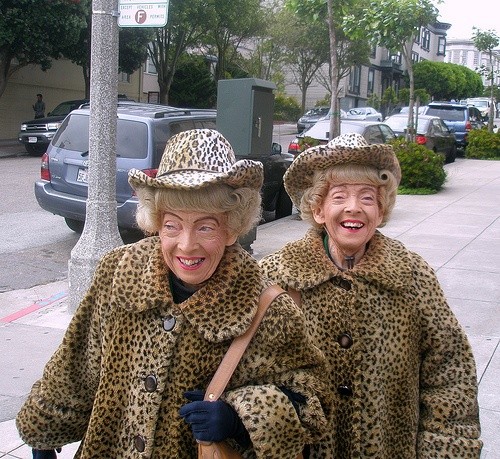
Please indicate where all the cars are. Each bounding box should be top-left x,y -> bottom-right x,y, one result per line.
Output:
297,107 -> 347,134
288,119 -> 398,160
347,107 -> 382,122
382,114 -> 457,164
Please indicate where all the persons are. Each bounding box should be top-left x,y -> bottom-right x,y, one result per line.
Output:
33,93 -> 46,118
15,130 -> 335,459
257,132 -> 483,459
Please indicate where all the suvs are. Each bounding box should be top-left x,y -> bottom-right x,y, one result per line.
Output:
18,94 -> 136,154
34,100 -> 296,236
400,97 -> 500,153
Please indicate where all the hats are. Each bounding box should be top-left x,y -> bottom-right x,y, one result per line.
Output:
127,128 -> 264,191
282,135 -> 401,210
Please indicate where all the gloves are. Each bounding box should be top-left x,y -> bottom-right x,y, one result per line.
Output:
32,443 -> 61,459
179,390 -> 242,443
